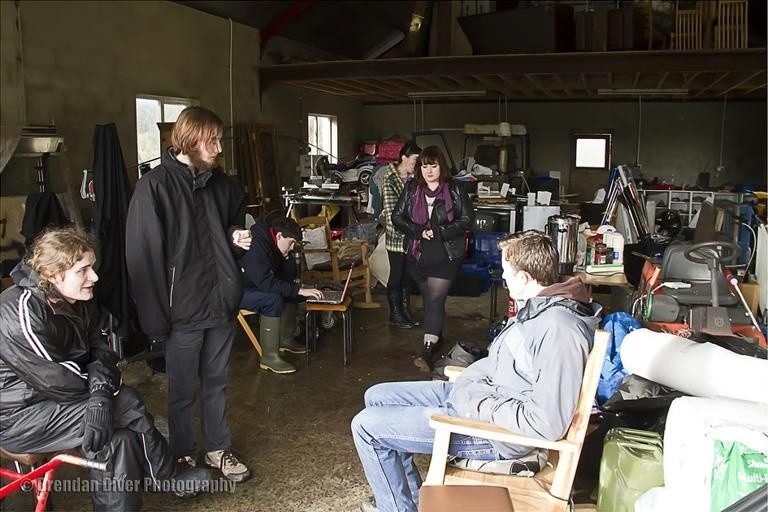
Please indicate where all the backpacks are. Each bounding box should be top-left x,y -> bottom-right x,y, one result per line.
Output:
597,312 -> 642,406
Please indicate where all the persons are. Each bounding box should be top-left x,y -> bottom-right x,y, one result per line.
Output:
0,228 -> 211,512
125,106 -> 252,499
351,229 -> 603,512
382,143 -> 423,329
239,217 -> 325,374
391,146 -> 476,373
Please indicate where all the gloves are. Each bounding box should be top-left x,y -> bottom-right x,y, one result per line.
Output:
144,340 -> 166,373
76,397 -> 115,453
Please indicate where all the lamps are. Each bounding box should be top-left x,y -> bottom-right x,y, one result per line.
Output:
597,87 -> 689,96
407,90 -> 487,98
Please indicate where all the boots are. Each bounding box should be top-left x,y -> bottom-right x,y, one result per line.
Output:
387,287 -> 419,328
260,304 -> 306,373
414,334 -> 439,373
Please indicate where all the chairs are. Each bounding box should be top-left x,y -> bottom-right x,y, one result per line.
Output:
235,309 -> 262,359
421,297 -> 611,512
290,205 -> 382,308
660,244 -> 738,329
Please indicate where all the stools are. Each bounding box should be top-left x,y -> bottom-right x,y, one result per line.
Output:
0,448 -> 64,512
419,484 -> 514,512
301,294 -> 354,367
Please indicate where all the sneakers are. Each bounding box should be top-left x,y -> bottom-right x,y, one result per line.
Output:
148,450 -> 251,497
360,497 -> 376,512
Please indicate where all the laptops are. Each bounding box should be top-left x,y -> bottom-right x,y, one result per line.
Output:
305,261 -> 355,305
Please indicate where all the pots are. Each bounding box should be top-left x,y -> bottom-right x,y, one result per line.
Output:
547,211 -> 579,274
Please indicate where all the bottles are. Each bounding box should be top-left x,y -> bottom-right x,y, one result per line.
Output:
578,221 -> 625,266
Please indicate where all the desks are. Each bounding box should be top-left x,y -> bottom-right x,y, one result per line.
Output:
572,271 -> 628,297
470,201 -> 521,235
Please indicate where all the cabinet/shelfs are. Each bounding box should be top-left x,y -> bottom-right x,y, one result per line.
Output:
636,188 -> 739,230
